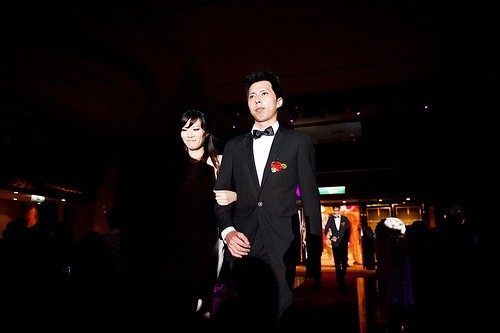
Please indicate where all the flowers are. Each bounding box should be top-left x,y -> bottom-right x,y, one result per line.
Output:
341,219 -> 346,226
269,158 -> 287,173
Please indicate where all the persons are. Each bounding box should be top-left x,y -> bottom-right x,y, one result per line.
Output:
1,180 -> 175,333
210,66 -> 321,333
160,109 -> 239,333
299,199 -> 500,333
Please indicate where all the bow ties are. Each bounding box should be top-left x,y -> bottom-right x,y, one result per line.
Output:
334,216 -> 339,218
253,126 -> 274,139
